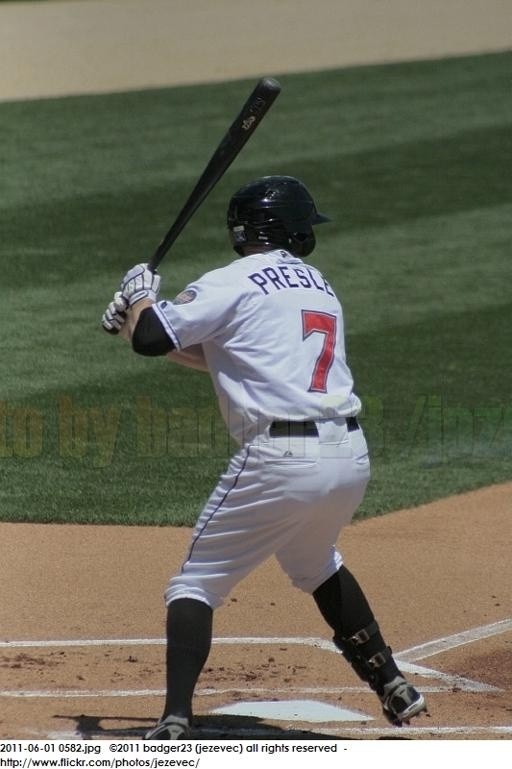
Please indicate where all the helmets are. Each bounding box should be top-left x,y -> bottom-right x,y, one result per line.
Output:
227,175 -> 333,258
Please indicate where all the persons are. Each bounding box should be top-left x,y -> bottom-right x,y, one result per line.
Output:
101,171 -> 426,740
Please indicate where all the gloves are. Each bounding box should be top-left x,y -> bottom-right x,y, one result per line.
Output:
101,262 -> 162,336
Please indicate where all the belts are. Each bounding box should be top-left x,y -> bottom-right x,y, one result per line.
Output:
268,416 -> 359,438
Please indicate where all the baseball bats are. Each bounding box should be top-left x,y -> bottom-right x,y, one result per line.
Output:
102,77 -> 280,335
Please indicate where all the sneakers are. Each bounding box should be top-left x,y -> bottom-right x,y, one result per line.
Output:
379,675 -> 427,726
144,714 -> 192,740
